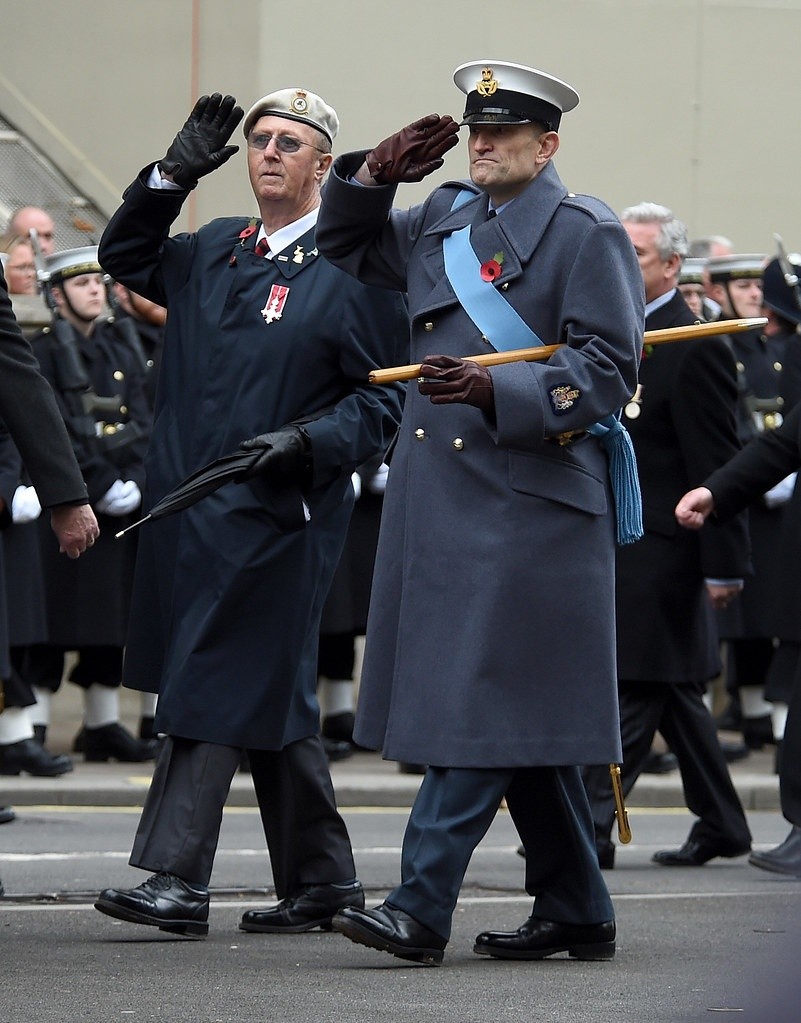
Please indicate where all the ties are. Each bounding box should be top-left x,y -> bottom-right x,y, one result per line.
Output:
485,210 -> 496,221
254,238 -> 271,256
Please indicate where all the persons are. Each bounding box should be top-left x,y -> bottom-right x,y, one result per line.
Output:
97,86 -> 410,938
314,59 -> 650,969
0,197 -> 801,880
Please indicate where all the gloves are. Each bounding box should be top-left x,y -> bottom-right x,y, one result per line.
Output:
233,427 -> 312,487
11,485 -> 41,524
93,478 -> 143,517
417,355 -> 495,413
160,92 -> 245,189
365,113 -> 460,186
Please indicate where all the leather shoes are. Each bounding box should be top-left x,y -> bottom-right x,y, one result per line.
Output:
73,710 -> 380,772
94,872 -> 210,936
517,832 -> 615,868
332,900 -> 447,967
473,914 -> 616,961
0,739 -> 73,776
651,825 -> 751,865
239,883 -> 365,934
713,708 -> 768,761
749,825 -> 801,879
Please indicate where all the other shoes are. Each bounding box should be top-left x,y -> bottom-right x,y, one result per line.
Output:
643,751 -> 676,773
0,808 -> 15,823
398,761 -> 426,775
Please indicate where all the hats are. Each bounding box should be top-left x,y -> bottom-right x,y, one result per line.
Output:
242,86 -> 340,148
677,258 -> 708,284
453,59 -> 580,134
44,245 -> 104,284
708,254 -> 769,281
763,234 -> 801,323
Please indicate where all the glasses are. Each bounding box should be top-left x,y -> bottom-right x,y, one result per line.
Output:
246,132 -> 325,154
